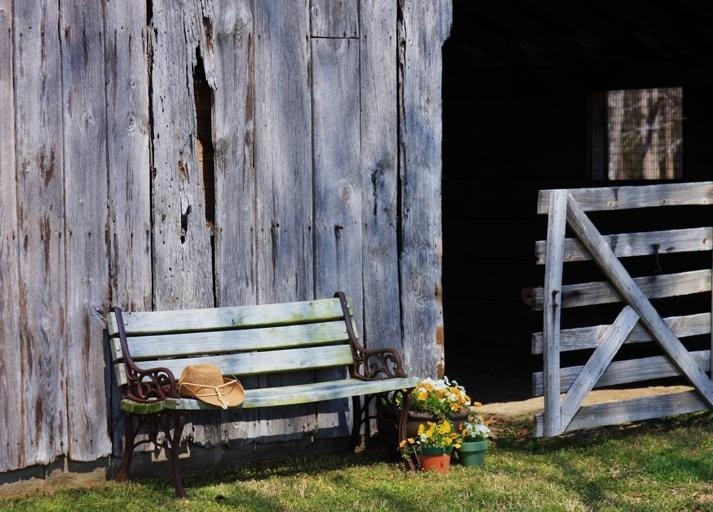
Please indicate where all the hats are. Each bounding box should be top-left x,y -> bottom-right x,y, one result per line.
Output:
162,362 -> 246,410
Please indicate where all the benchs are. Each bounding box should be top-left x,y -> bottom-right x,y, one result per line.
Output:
102,288 -> 421,499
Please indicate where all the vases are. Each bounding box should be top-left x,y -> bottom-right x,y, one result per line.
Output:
415,439 -> 490,475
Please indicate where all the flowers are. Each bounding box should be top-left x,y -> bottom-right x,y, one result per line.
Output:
398,372 -> 496,448
